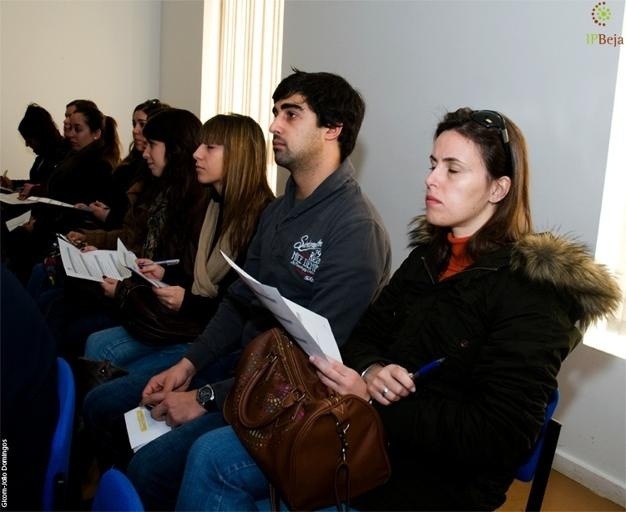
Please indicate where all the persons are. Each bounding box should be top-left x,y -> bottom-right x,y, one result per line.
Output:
1,97 -> 211,378
176,104 -> 622,511
81,70 -> 392,511
44,111 -> 276,486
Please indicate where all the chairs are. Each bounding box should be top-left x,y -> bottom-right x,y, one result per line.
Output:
43,355 -> 78,512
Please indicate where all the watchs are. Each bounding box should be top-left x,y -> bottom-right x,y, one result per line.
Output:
193,381 -> 218,415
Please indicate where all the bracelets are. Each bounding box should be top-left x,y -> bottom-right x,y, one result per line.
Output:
358,361 -> 380,379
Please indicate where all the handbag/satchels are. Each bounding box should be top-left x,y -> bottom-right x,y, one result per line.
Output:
122,293 -> 203,347
225,326 -> 391,511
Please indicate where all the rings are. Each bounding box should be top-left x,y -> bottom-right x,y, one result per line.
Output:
380,386 -> 390,397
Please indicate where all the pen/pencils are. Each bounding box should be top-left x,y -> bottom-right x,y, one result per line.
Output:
139,259 -> 179,266
2,170 -> 7,178
411,358 -> 445,380
104,208 -> 110,209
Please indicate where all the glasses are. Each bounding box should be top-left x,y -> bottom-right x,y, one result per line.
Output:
145,98 -> 161,108
433,110 -> 512,173
